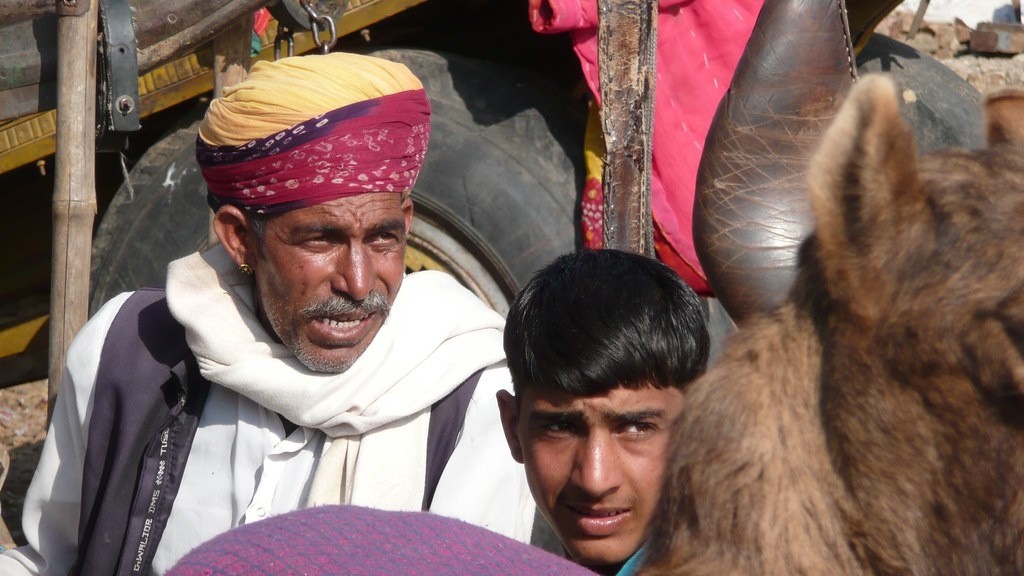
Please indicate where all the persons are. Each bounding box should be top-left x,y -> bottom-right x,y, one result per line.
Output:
494,247 -> 712,575
1,49 -> 573,576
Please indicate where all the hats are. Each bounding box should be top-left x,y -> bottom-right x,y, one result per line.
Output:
196,52 -> 432,219
164,506 -> 601,576
